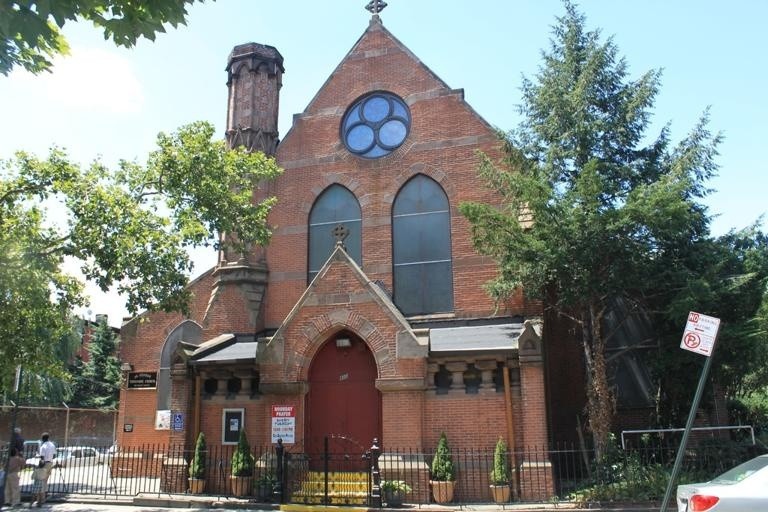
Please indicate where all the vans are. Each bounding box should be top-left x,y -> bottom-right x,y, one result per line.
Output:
2,440 -> 54,467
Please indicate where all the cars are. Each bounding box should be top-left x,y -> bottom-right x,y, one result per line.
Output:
25,447 -> 105,467
95,442 -> 118,454
675,453 -> 767,512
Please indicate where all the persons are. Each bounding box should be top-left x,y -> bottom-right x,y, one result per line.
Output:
28,432 -> 57,509
4,428 -> 26,507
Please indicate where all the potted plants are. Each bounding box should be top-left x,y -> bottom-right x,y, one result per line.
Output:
427,432 -> 456,504
230,426 -> 255,499
255,469 -> 277,499
381,478 -> 411,506
187,432 -> 207,494
489,437 -> 511,504
